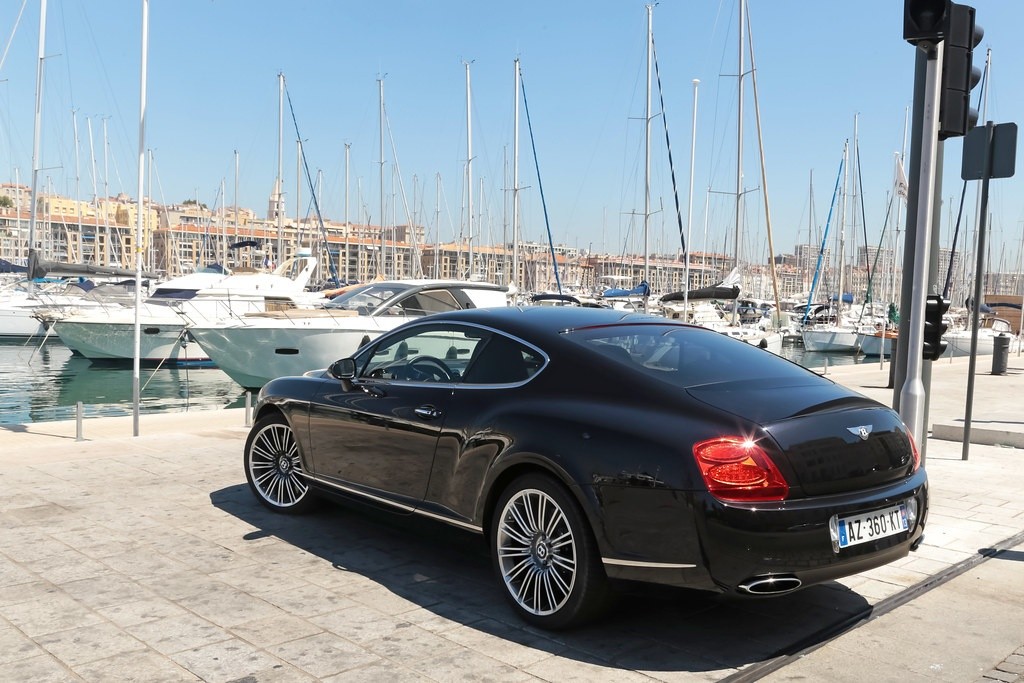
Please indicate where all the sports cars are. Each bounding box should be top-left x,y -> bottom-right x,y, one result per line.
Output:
240,306 -> 935,631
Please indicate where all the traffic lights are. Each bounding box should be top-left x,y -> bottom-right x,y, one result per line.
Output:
902,0 -> 949,41
940,4 -> 985,142
924,295 -> 951,361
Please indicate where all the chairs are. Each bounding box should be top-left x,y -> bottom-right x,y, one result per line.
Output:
463,340 -> 529,386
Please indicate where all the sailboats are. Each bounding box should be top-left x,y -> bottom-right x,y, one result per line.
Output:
0,0 -> 1024,391
